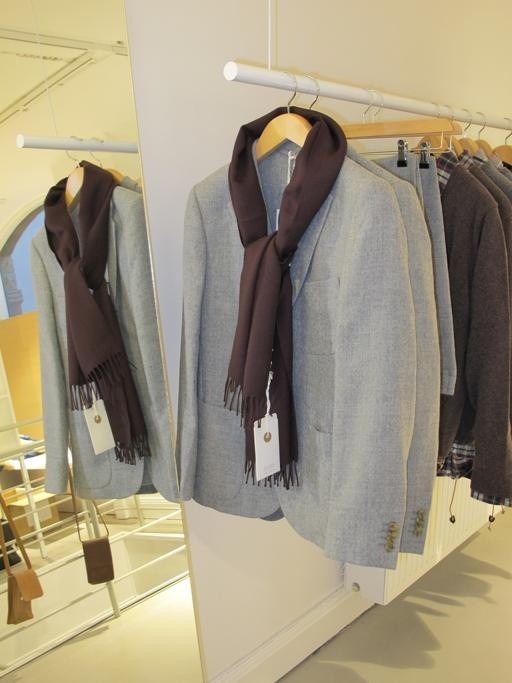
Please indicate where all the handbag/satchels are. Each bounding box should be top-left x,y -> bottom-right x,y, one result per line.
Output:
7,569 -> 43,625
83,537 -> 114,585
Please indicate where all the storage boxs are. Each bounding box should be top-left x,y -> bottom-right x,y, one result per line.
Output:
3,489 -> 60,537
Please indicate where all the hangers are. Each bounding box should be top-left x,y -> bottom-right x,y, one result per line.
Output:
64,135 -> 143,208
250,68 -> 511,169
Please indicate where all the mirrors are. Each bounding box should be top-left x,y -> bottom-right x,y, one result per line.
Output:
0,0 -> 204,683
